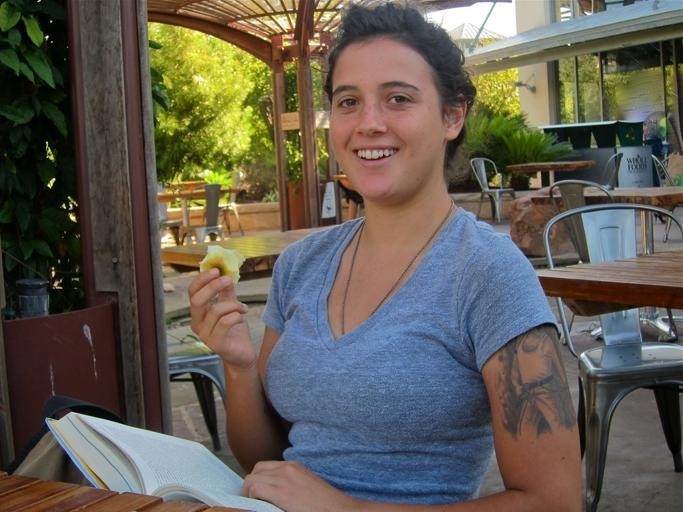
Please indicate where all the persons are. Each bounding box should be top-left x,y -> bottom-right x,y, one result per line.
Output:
185,5 -> 584,511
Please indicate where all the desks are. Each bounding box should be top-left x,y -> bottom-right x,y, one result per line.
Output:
527,182 -> 682,254
535,248 -> 683,319
506,160 -> 682,343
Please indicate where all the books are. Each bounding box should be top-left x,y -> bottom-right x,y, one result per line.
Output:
40,411 -> 282,511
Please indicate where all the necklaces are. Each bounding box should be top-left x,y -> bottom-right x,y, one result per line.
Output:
338,195 -> 455,341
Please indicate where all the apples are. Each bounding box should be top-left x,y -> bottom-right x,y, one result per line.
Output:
199,244 -> 244,285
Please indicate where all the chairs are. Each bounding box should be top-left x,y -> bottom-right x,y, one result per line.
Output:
602,153 -> 675,244
157,172 -> 245,246
470,157 -> 517,224
546,177 -> 618,260
544,202 -> 680,509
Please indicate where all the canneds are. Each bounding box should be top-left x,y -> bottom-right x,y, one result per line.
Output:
16,278 -> 51,317
